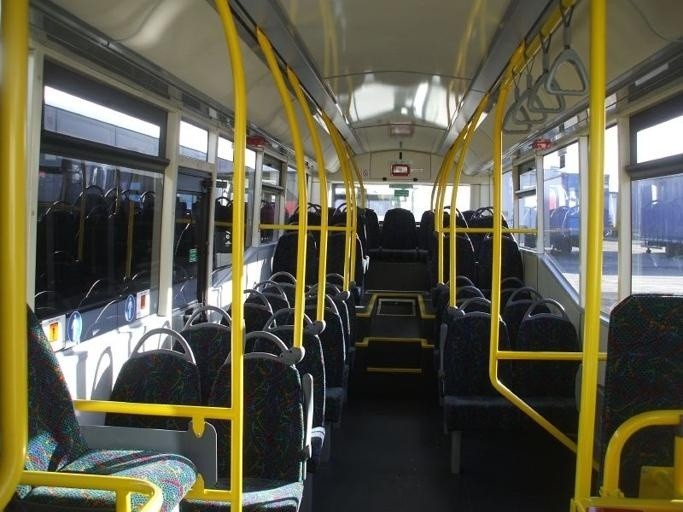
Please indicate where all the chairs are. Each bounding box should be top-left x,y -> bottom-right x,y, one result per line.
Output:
420,206 -> 524,315
380,207 -> 417,263
433,276 -> 582,498
600,292 -> 683,497
9,304 -> 197,510
106,271 -> 373,512
273,203 -> 379,314
